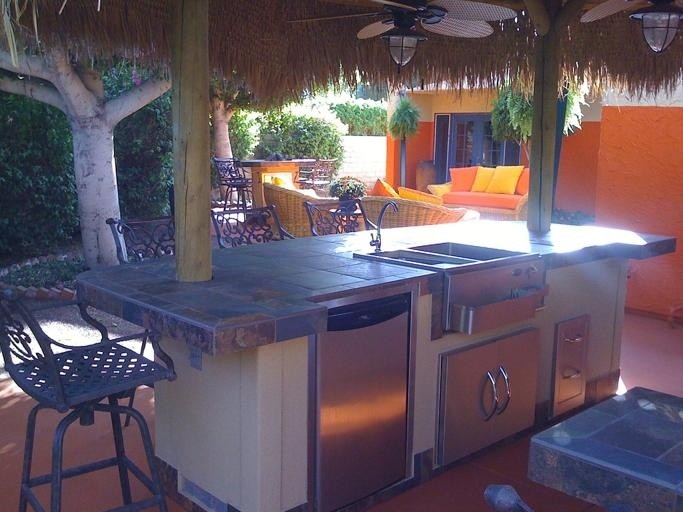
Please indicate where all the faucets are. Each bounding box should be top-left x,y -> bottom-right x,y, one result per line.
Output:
370,202 -> 399,250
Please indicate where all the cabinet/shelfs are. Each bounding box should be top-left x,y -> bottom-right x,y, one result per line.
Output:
436,327 -> 537,471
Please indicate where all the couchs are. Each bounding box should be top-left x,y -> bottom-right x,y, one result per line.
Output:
429,164 -> 531,224
362,190 -> 468,228
266,176 -> 339,237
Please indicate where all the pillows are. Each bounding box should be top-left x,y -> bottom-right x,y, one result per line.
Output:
368,178 -> 400,200
447,166 -> 480,194
398,186 -> 445,204
472,167 -> 495,194
489,167 -> 524,195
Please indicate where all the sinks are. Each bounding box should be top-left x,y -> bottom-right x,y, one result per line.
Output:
409,241 -> 539,263
352,249 -> 481,271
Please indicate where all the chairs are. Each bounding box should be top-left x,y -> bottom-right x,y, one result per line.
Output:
211,203 -> 297,250
216,157 -> 255,225
2,280 -> 177,507
105,215 -> 176,265
299,159 -> 337,195
302,198 -> 380,235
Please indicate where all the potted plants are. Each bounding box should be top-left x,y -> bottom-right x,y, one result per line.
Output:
332,175 -> 367,211
